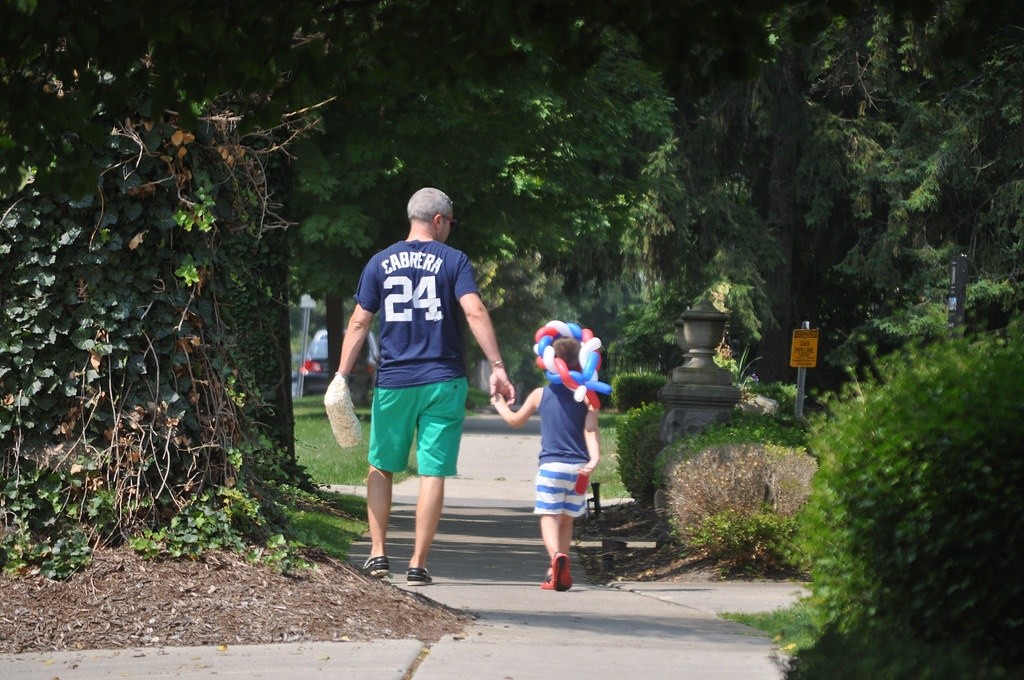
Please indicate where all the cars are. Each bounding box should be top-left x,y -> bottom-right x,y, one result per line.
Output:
294,326 -> 378,393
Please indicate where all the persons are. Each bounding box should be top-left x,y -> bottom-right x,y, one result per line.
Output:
490,337 -> 600,593
324,188 -> 516,586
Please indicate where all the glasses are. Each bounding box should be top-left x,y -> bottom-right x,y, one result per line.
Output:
432,214 -> 458,228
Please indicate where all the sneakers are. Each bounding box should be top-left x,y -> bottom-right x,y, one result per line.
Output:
541,569 -> 554,590
552,552 -> 572,591
363,555 -> 390,576
406,567 -> 431,586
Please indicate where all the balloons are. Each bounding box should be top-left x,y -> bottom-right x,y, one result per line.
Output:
534,321 -> 612,408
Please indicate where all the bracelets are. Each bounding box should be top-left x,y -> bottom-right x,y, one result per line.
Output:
334,371 -> 350,382
490,360 -> 504,368
